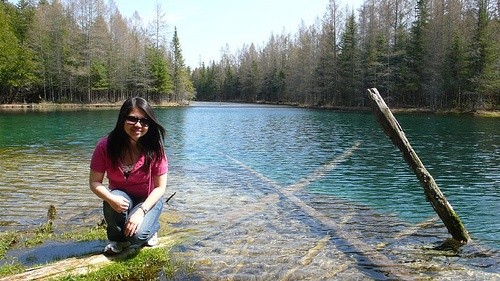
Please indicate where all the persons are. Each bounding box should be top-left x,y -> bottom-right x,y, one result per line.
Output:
89,97 -> 168,255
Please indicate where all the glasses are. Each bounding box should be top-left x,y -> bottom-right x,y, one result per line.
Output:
125,116 -> 150,127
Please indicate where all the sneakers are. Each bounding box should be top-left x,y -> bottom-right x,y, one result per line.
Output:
104,241 -> 131,253
145,232 -> 158,246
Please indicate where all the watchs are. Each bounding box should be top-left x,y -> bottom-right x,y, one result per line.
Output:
139,204 -> 148,214
